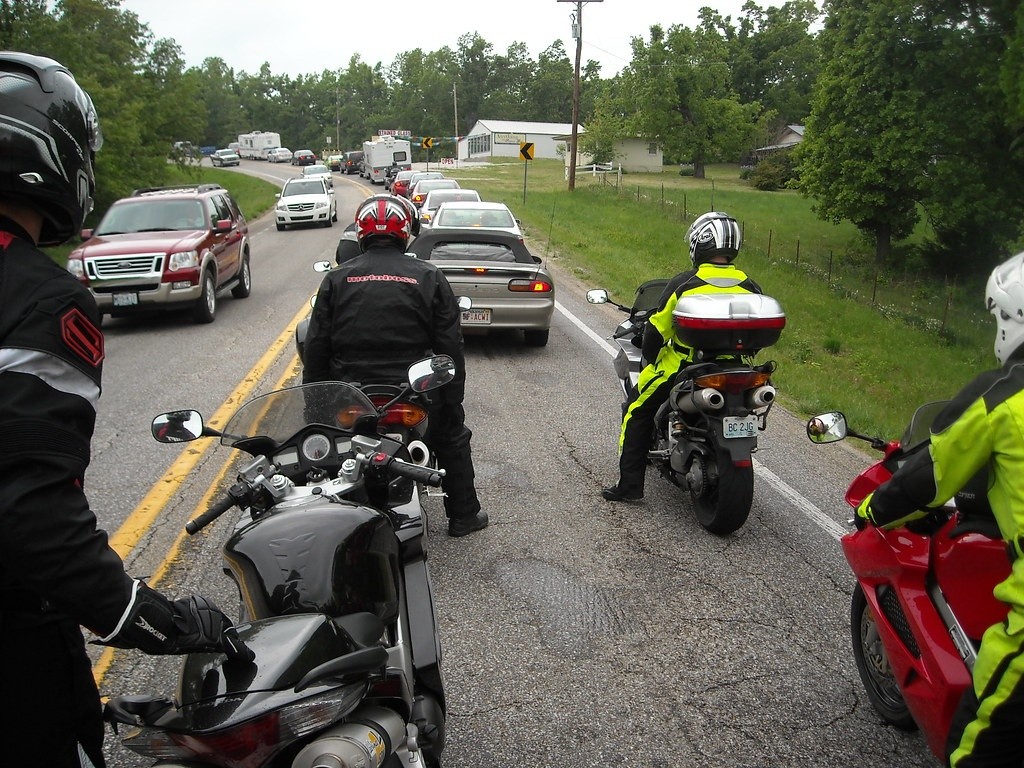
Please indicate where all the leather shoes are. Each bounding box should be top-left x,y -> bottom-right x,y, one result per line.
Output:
448,510 -> 487,537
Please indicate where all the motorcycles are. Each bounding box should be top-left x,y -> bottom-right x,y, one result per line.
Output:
308,253 -> 471,466
96,349 -> 465,767
807,397 -> 1016,768
584,282 -> 781,536
383,167 -> 403,190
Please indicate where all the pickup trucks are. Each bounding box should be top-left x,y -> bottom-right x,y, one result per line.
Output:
209,149 -> 240,166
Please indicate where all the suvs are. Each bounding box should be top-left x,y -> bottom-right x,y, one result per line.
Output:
68,183 -> 251,324
274,176 -> 338,230
339,151 -> 365,175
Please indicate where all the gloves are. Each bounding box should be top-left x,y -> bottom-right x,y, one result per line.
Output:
87,577 -> 240,656
854,489 -> 875,532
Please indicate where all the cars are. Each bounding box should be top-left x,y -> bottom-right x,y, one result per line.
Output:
422,201 -> 525,245
405,229 -> 555,347
291,150 -> 316,166
389,170 -> 444,195
409,179 -> 462,208
267,147 -> 293,163
227,142 -> 239,156
418,190 -> 482,225
323,155 -> 343,171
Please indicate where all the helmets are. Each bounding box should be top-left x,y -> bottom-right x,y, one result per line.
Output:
683,212 -> 741,269
984,252 -> 1024,366
0,50 -> 104,248
354,194 -> 413,255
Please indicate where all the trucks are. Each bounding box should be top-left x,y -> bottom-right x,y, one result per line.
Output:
357,135 -> 412,183
174,141 -> 192,156
238,131 -> 281,160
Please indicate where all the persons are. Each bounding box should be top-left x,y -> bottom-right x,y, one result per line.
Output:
602,210 -> 763,503
152,411 -> 196,441
410,357 -> 455,390
0,50 -> 286,768
301,194 -> 489,537
809,418 -> 839,443
852,251 -> 1024,767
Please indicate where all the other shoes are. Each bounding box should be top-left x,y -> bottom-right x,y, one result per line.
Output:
602,484 -> 644,502
